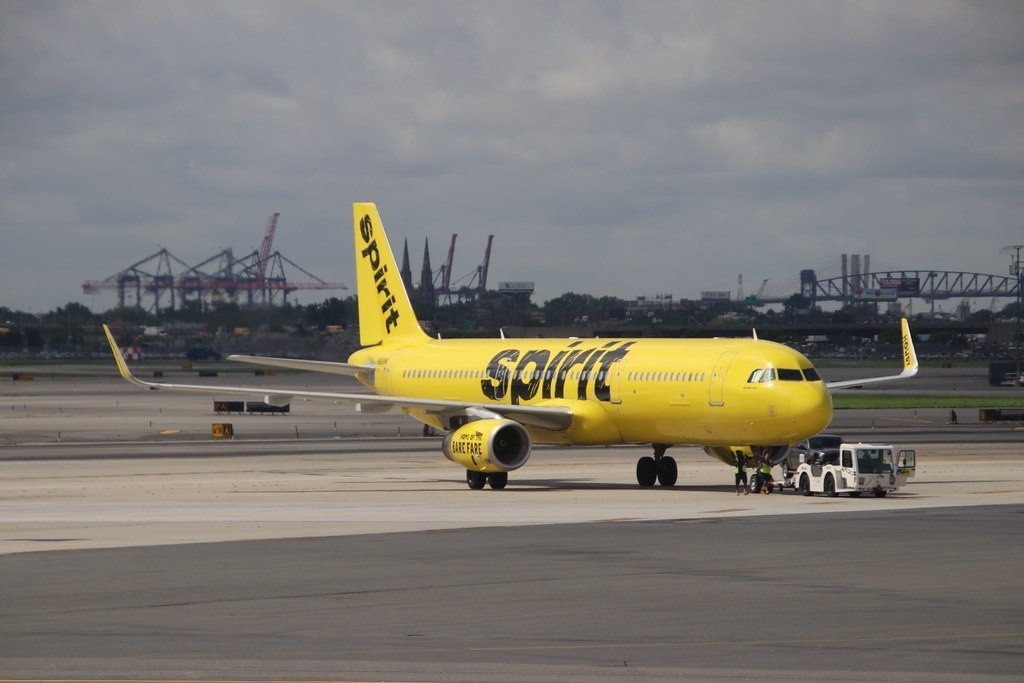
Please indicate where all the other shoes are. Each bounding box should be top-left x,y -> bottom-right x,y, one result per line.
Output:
743,489 -> 748,495
736,490 -> 739,495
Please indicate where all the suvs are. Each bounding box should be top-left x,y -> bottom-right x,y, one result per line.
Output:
779,436 -> 844,480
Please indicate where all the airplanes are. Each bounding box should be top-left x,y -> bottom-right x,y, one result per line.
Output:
103,202 -> 918,490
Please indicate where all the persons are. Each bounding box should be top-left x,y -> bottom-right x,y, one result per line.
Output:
734,451 -> 749,495
755,454 -> 773,495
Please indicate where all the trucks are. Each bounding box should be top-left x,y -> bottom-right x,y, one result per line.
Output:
796,444 -> 916,497
